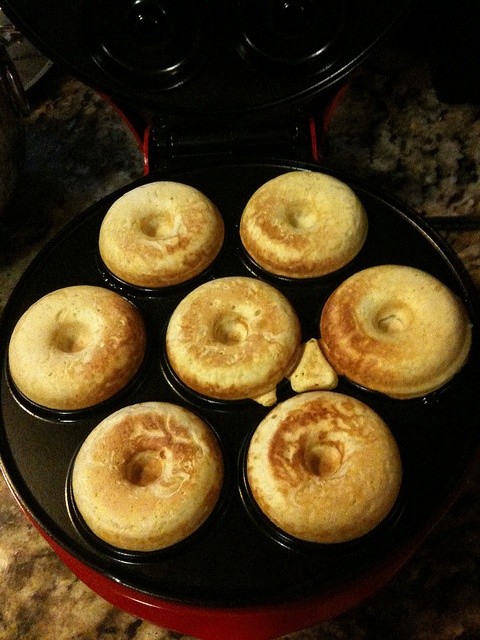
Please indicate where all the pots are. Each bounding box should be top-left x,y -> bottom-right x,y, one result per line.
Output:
3,2 -> 477,632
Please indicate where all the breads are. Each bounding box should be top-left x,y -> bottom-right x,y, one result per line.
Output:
71,400 -> 221,552
320,264 -> 470,398
8,285 -> 144,409
99,180 -> 224,289
246,389 -> 403,545
239,169 -> 368,277
166,275 -> 301,400
